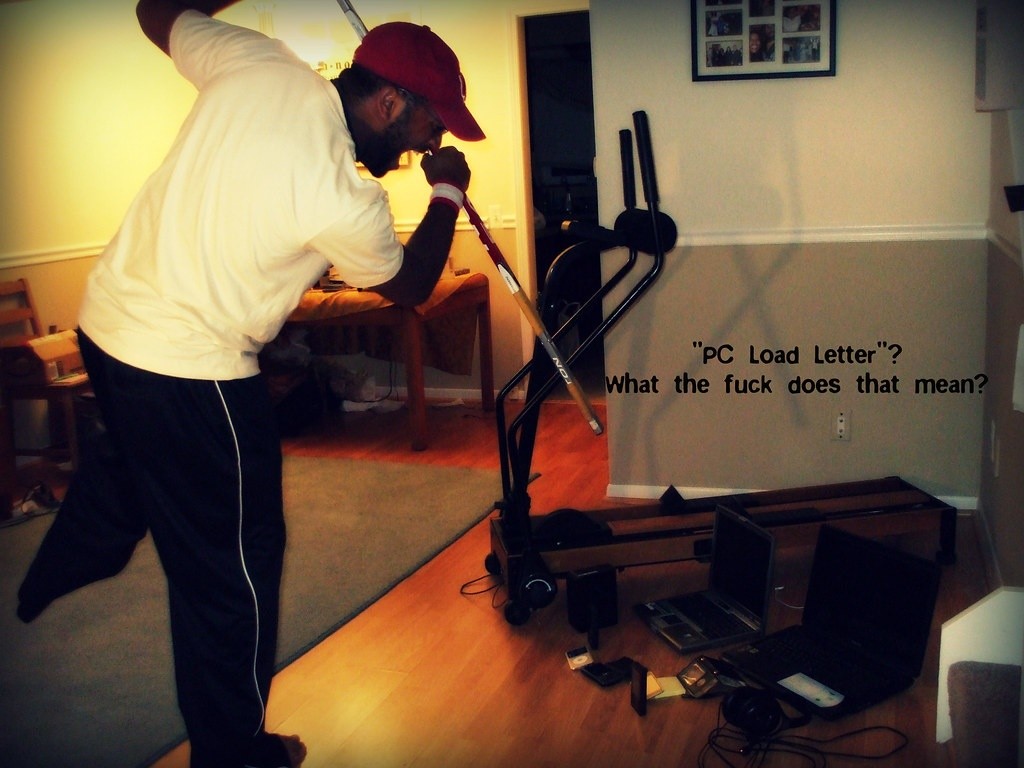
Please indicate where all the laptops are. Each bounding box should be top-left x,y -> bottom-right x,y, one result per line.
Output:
631,503 -> 776,654
720,522 -> 942,721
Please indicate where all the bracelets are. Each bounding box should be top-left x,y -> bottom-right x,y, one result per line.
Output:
430,182 -> 464,209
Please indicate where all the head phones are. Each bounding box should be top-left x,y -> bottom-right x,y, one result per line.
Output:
721,685 -> 813,738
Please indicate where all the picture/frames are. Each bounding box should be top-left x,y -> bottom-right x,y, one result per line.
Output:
690,0 -> 837,82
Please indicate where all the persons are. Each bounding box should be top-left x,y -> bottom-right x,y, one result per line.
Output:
19,1 -> 486,767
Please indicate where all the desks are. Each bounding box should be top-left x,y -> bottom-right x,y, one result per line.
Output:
277,271 -> 495,451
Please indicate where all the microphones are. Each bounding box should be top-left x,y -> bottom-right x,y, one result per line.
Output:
740,739 -> 762,756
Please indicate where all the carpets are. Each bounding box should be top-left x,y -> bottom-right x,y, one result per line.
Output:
0,453 -> 543,768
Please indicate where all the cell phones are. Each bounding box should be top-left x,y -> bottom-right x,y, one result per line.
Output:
582,662 -> 624,687
631,662 -> 647,715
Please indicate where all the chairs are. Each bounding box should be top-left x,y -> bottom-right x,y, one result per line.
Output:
0,276 -> 91,507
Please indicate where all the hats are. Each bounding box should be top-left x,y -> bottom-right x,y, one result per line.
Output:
351,22 -> 486,144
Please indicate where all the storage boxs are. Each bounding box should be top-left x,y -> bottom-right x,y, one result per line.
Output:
0,330 -> 84,391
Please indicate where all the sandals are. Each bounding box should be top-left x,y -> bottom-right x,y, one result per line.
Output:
187,729 -> 310,768
